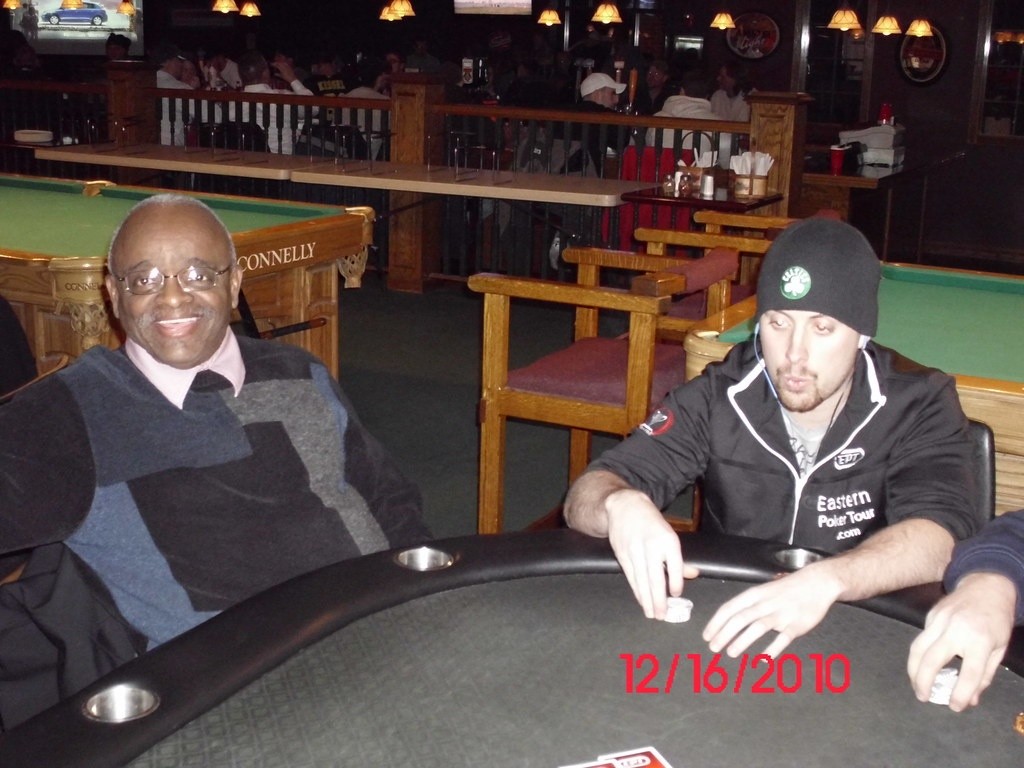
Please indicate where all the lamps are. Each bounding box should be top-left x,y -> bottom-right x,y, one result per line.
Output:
205,0 -> 933,42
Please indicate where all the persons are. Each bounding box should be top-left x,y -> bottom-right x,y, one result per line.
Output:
711,60 -> 754,156
103,26 -> 458,163
0,194 -> 434,734
0,3 -> 102,179
562,218 -> 980,663
440,37 -> 712,183
907,509 -> 1024,714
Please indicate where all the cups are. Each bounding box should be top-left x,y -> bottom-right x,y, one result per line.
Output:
829,147 -> 845,175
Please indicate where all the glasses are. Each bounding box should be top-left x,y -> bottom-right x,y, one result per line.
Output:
645,71 -> 665,80
106,262 -> 232,296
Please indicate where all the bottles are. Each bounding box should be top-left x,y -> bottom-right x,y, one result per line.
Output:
879,102 -> 893,121
679,175 -> 692,199
235,80 -> 240,91
223,81 -> 228,90
662,175 -> 675,196
699,174 -> 714,201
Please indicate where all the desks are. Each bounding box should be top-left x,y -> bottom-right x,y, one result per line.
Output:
2,167 -> 375,394
0,527 -> 997,768
802,147 -> 906,264
36,142 -> 660,210
680,253 -> 1024,513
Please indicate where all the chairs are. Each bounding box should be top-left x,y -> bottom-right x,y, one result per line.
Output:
631,205 -> 842,345
466,247 -> 739,539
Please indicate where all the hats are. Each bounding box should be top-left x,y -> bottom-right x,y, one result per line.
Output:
580,73 -> 626,98
753,217 -> 882,338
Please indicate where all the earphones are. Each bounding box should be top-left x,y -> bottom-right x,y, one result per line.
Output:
754,321 -> 760,333
861,340 -> 867,352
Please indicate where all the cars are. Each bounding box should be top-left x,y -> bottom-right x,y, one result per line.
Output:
40,1 -> 108,27
